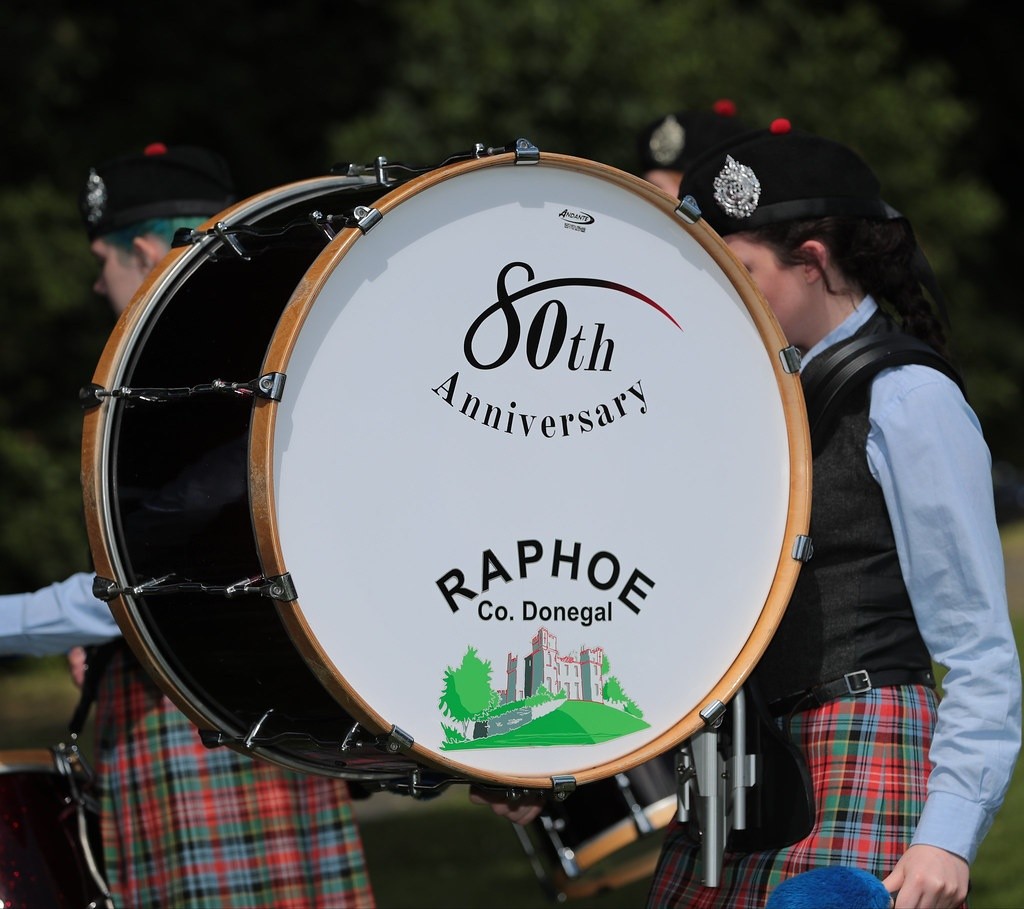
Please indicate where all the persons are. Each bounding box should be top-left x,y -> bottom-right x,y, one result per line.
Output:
638,98 -> 749,202
467,128 -> 1023,908
0,138 -> 374,909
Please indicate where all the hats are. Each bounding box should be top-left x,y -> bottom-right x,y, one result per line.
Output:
630,99 -> 741,171
78,141 -> 227,241
678,121 -> 881,236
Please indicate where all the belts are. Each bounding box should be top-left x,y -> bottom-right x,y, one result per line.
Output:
769,669 -> 936,719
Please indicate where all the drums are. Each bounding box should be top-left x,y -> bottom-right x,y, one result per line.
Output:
509,750 -> 684,898
1,744 -> 128,909
69,138 -> 823,799
